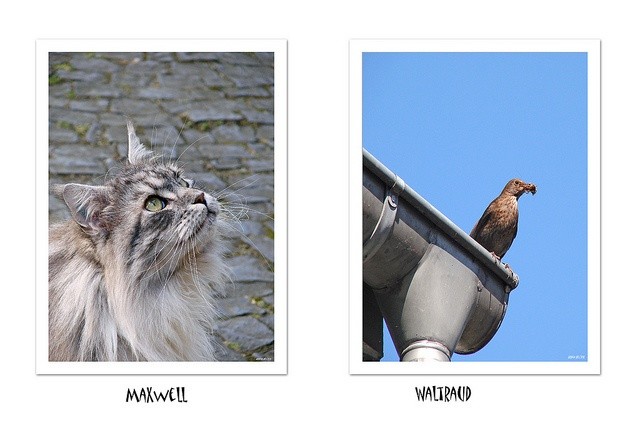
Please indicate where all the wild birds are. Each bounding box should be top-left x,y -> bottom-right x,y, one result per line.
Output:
469,179 -> 538,277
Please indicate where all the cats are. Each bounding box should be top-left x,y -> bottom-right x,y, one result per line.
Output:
49,118 -> 228,361
